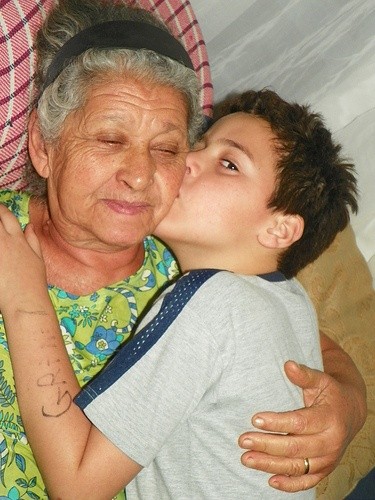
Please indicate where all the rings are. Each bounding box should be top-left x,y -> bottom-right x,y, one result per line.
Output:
304,457 -> 310,474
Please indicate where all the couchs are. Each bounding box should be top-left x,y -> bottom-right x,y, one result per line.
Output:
1,0 -> 374,497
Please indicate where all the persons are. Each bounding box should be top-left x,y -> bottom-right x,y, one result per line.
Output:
1,85 -> 357,499
0,1 -> 366,498
